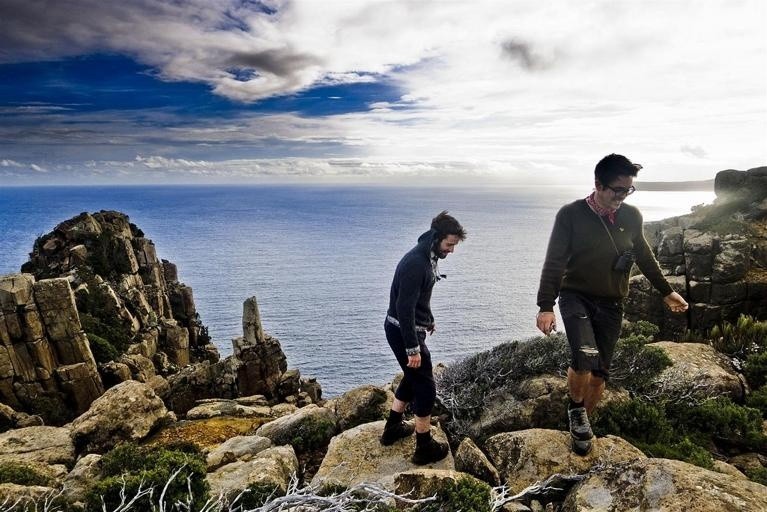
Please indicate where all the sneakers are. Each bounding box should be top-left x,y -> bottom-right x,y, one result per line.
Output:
567,403 -> 596,456
381,420 -> 416,446
411,436 -> 449,465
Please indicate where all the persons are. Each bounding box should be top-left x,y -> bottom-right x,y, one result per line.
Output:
537,153 -> 690,455
380,209 -> 466,464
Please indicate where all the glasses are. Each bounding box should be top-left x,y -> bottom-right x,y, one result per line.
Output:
605,183 -> 635,198
